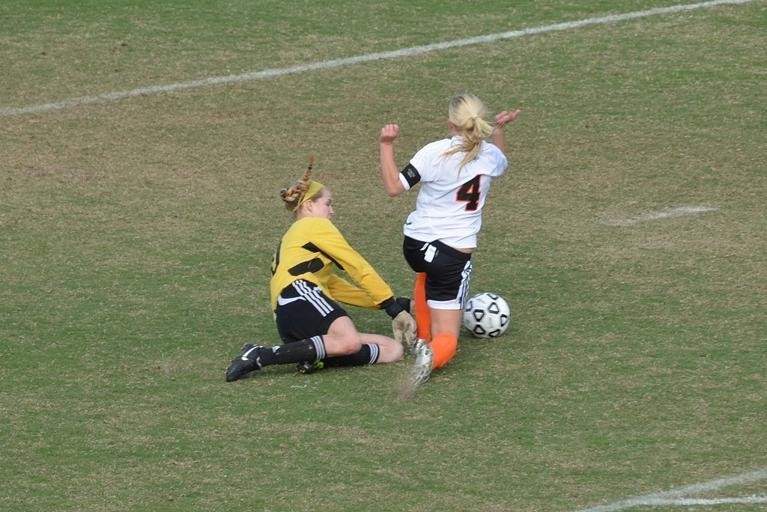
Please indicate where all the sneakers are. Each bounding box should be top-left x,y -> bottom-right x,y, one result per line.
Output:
226,343 -> 265,382
295,359 -> 327,374
413,339 -> 433,386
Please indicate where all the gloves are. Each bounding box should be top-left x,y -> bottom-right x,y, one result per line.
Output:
392,296 -> 418,352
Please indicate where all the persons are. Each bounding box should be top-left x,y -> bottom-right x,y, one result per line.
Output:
379,93 -> 521,385
226,154 -> 418,382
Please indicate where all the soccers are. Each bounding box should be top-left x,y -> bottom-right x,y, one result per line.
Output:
464,291 -> 511,337
